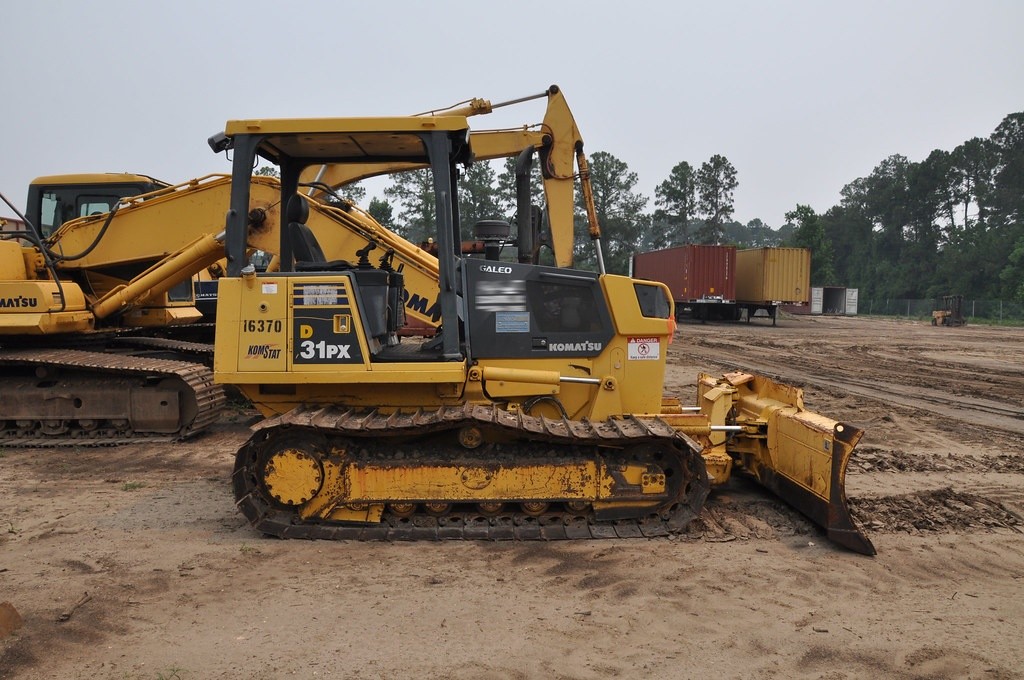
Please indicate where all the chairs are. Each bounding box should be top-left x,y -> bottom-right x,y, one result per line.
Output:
285,192 -> 357,271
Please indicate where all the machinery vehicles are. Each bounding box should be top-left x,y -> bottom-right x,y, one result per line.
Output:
931,295 -> 967,327
207,85 -> 880,557
0,174 -> 439,446
